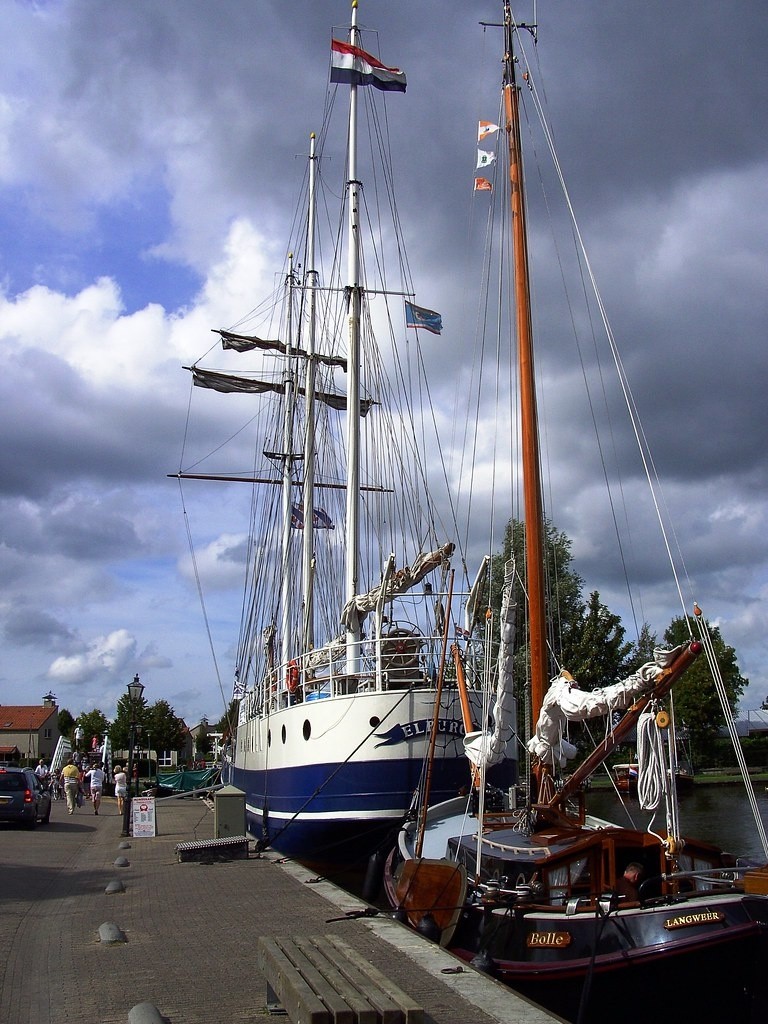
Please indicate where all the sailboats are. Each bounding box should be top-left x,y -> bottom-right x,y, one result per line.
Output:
166,0 -> 522,887
381,0 -> 768,1010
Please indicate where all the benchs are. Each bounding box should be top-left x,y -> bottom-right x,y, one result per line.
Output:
177,836 -> 250,863
257,933 -> 423,1024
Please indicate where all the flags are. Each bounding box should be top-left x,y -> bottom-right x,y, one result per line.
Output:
474,178 -> 492,191
291,501 -> 335,530
457,627 -> 470,641
479,121 -> 499,141
629,766 -> 639,777
330,39 -> 407,94
476,148 -> 495,169
405,299 -> 443,336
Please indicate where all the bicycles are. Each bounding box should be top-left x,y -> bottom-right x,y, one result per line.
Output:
50,774 -> 59,801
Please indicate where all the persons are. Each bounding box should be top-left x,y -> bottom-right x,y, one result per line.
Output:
82,750 -> 90,764
614,862 -> 643,903
72,748 -> 82,764
133,764 -> 137,782
60,759 -> 79,815
51,764 -> 91,800
85,764 -> 104,815
113,765 -> 126,815
74,724 -> 84,747
34,759 -> 48,776
91,734 -> 98,748
186,758 -> 206,770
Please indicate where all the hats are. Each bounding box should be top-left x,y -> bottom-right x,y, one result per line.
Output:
113,765 -> 121,772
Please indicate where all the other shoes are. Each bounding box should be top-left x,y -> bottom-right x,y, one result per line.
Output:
68,810 -> 73,815
54,797 -> 57,800
120,812 -> 123,815
94,811 -> 98,815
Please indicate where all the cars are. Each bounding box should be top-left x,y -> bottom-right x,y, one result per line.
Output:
0,766 -> 52,829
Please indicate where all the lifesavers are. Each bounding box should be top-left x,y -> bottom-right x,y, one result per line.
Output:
285,660 -> 300,692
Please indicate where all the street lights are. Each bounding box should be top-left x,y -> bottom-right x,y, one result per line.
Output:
145,729 -> 155,780
135,722 -> 142,797
118,672 -> 145,839
